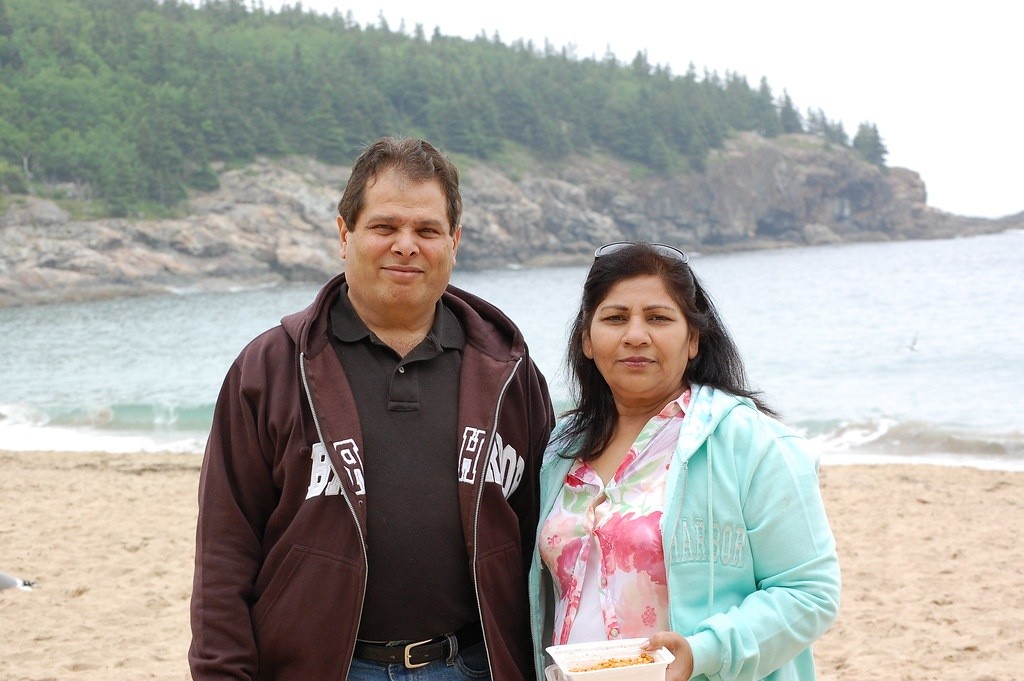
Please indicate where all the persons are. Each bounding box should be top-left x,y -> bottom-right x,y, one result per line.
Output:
188,137 -> 557,681
523,240 -> 843,681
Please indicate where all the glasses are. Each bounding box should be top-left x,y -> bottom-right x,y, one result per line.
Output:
594,239 -> 690,268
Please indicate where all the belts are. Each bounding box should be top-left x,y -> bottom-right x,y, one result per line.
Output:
353,621 -> 484,669
